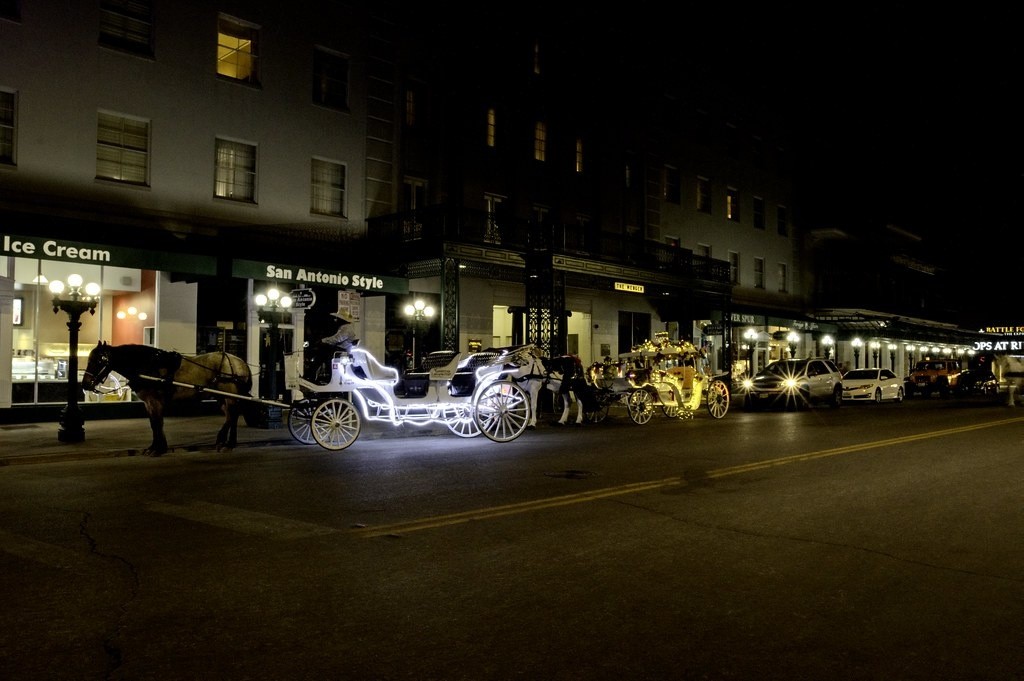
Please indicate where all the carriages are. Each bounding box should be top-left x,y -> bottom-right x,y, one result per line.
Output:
81,335 -> 536,457
509,332 -> 731,431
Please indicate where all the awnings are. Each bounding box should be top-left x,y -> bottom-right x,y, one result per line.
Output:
723,301 -> 838,333
227,253 -> 409,294
0,223 -> 216,276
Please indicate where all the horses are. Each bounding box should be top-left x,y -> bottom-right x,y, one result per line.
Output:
81,340 -> 253,457
508,352 -> 589,430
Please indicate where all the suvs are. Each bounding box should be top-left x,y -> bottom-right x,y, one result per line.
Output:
907,359 -> 963,400
744,357 -> 844,413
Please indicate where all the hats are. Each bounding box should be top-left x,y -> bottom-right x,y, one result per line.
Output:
330,307 -> 353,323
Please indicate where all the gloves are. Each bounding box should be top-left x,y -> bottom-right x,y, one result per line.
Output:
314,339 -> 322,345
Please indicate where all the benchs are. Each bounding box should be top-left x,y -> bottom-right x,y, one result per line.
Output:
350,350 -> 399,385
449,351 -> 506,397
401,351 -> 460,397
665,366 -> 695,400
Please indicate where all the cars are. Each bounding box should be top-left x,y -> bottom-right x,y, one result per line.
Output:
840,367 -> 906,406
961,367 -> 1000,397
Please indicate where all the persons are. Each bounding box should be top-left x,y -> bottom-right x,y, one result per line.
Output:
322,308 -> 359,348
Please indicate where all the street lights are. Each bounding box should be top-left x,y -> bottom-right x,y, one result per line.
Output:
400,298 -> 436,373
919,346 -> 929,361
871,342 -> 881,369
744,327 -> 758,379
820,335 -> 835,360
254,287 -> 293,429
49,265 -> 101,446
787,331 -> 799,358
888,343 -> 898,373
906,344 -> 915,373
851,338 -> 862,369
